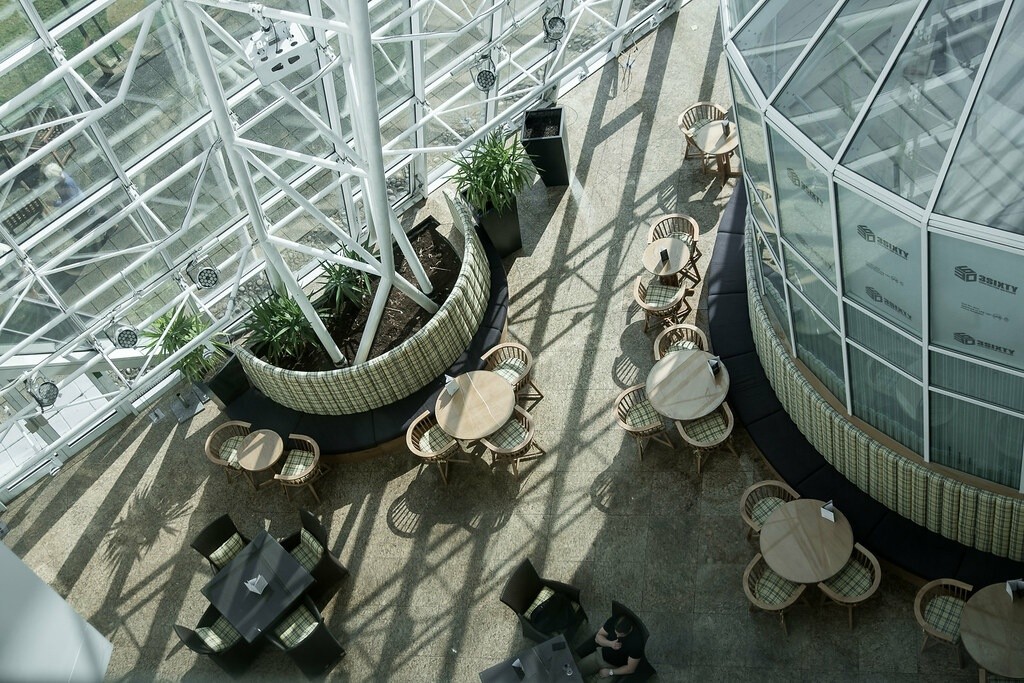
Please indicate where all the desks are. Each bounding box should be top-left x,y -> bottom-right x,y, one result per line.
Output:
760,500 -> 853,584
960,582 -> 1024,680
697,119 -> 743,190
645,350 -> 729,421
479,634 -> 584,683
434,370 -> 514,440
641,238 -> 694,313
238,428 -> 284,490
200,529 -> 314,644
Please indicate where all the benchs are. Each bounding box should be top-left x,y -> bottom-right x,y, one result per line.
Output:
703,172 -> 1023,591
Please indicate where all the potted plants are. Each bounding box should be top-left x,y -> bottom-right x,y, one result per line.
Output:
137,305 -> 252,420
443,121 -> 546,259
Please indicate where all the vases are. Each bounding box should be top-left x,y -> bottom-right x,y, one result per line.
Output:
520,108 -> 571,187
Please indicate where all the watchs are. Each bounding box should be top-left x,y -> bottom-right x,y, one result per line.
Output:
609,669 -> 613,675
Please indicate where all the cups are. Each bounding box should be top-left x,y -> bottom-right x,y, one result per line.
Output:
563,663 -> 573,676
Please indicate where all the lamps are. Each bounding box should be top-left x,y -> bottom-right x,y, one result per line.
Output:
185,253 -> 222,291
102,315 -> 139,349
27,369 -> 60,408
469,52 -> 498,91
543,2 -> 566,45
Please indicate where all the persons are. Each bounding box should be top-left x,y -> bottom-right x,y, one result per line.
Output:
44,163 -> 118,252
579,616 -> 644,683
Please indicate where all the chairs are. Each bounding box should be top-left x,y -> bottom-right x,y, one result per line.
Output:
614,212 -> 887,640
406,344 -> 546,487
205,420 -> 337,506
579,600 -> 657,683
677,101 -> 735,172
914,578 -> 1024,683
173,514 -> 350,681
0,106 -> 76,235
499,559 -> 588,648
